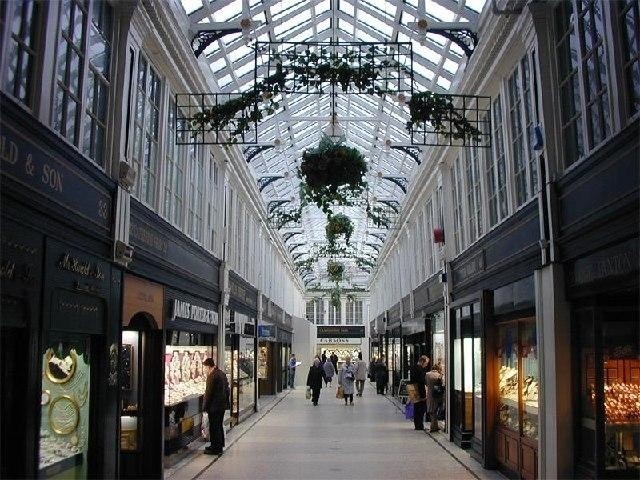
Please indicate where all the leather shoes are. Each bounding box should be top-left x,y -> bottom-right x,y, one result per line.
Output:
203,446 -> 225,456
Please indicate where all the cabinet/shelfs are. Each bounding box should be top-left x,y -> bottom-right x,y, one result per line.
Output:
494,310 -> 539,480
224,334 -> 256,425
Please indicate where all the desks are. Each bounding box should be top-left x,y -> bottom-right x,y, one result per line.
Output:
576,416 -> 640,470
164,393 -> 208,468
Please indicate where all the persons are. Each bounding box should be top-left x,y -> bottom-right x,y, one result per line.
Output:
354,352 -> 366,396
202,358 -> 230,454
307,350 -> 338,406
369,354 -> 388,395
289,354 -> 299,388
410,355 -> 445,432
338,356 -> 354,405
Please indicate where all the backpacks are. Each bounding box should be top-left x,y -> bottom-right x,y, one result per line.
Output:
432,375 -> 444,398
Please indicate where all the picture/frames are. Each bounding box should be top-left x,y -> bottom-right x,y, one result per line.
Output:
44,348 -> 76,383
47,395 -> 79,435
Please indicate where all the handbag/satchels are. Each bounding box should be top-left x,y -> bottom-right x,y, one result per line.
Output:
344,371 -> 354,380
336,385 -> 345,399
306,389 -> 311,400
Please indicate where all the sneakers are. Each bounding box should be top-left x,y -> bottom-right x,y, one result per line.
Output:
356,393 -> 362,397
